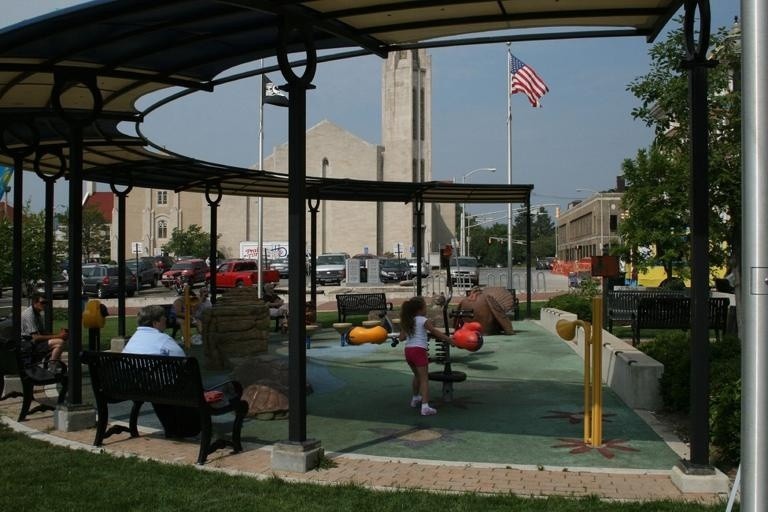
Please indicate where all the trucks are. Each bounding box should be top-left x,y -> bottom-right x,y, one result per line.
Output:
622,223 -> 727,291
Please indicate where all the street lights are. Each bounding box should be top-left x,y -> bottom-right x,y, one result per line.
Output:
460,167 -> 499,258
530,210 -> 558,256
575,188 -> 605,256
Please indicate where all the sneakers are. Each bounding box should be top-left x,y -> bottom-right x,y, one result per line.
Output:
410,399 -> 420,408
421,407 -> 437,416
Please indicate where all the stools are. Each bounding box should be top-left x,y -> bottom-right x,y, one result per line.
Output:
362,318 -> 380,330
333,322 -> 353,347
306,324 -> 318,349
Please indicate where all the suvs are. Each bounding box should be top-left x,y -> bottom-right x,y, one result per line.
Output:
445,255 -> 482,287
29,255 -> 206,298
315,252 -> 351,285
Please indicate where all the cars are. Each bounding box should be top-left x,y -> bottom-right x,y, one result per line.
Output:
270,260 -> 288,278
535,255 -> 559,270
350,252 -> 378,282
497,264 -> 503,268
406,256 -> 431,277
378,258 -> 414,283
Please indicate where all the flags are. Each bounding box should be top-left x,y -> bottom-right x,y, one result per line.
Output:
510,53 -> 549,109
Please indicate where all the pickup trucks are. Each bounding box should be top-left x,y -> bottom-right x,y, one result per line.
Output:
201,257 -> 281,290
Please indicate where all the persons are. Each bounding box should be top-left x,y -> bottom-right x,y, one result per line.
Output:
195,288 -> 213,320
20,291 -> 69,373
399,295 -> 455,416
262,281 -> 289,328
120,305 -> 203,443
168,290 -> 204,342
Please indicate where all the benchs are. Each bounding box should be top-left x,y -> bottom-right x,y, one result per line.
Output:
262,300 -> 288,332
631,297 -> 735,344
345,326 -> 388,345
77,348 -> 246,467
334,289 -> 394,338
0,332 -> 70,422
609,292 -> 684,333
